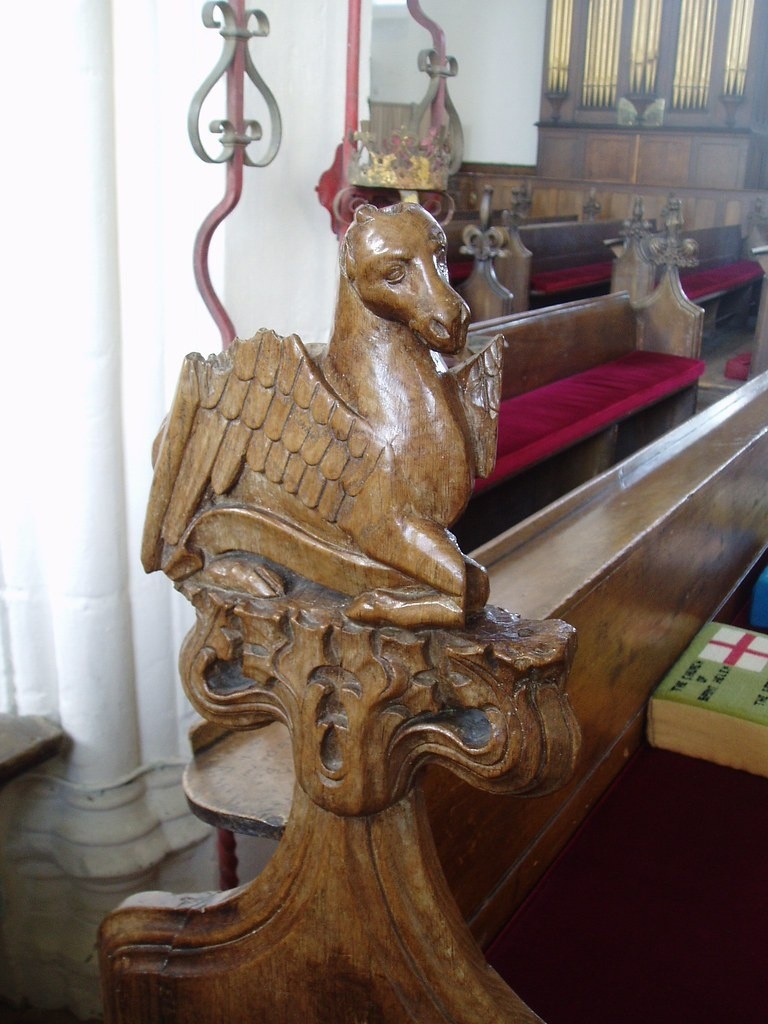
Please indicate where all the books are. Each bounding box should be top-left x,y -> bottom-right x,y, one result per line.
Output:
647,621 -> 768,778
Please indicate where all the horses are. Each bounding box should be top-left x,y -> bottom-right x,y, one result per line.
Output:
141,203 -> 505,631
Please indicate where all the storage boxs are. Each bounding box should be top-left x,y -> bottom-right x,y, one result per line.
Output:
647,624 -> 767,778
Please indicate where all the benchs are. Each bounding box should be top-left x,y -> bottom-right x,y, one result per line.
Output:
96,0 -> 768,1024
